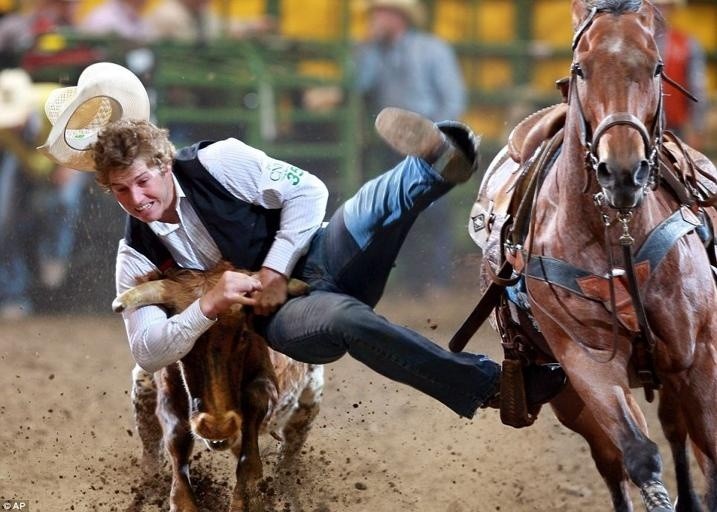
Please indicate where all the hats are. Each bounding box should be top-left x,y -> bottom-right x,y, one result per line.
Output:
36,61 -> 151,173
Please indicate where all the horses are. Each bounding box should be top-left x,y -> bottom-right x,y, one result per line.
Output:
477,1 -> 717,512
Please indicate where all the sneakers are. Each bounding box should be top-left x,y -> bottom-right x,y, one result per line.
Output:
374,106 -> 471,184
479,363 -> 565,409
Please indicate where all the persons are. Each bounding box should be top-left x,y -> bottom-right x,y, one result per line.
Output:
642,3 -> 711,151
144,1 -> 276,141
1,66 -> 92,326
88,106 -> 566,420
1,0 -> 82,78
298,0 -> 474,307
80,3 -> 160,151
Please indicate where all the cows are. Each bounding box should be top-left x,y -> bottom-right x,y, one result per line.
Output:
108,258 -> 321,512
